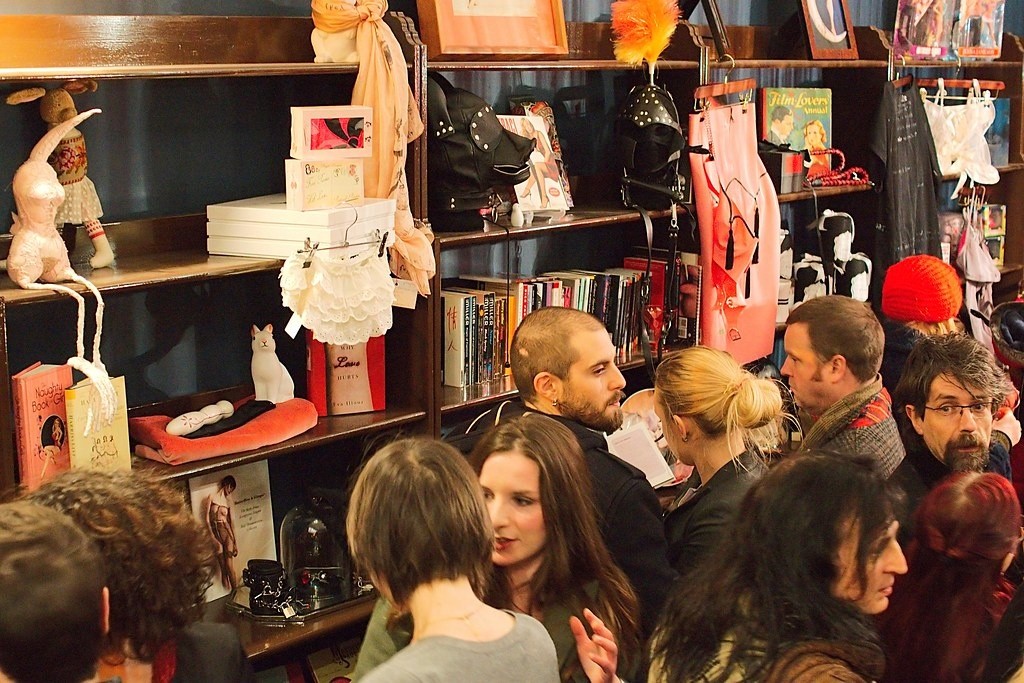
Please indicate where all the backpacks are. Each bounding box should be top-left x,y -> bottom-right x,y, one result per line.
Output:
423,69 -> 538,230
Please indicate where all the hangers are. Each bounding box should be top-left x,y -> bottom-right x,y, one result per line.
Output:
296,203 -> 391,269
891,53 -> 912,89
692,53 -> 756,112
913,50 -> 1005,100
956,178 -> 990,206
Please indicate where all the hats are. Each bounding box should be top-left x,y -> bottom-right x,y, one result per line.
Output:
609,0 -> 685,171
882,254 -> 964,324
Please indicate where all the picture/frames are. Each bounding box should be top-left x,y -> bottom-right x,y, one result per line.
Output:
798,0 -> 860,60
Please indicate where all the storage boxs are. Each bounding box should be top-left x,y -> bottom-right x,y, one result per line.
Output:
207,157 -> 397,261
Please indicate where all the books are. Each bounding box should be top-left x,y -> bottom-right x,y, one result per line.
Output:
440,246 -> 699,387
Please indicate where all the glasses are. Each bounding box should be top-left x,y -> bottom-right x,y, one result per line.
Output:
923,397 -> 1000,419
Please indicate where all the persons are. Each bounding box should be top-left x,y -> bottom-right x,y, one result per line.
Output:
346,437 -> 561,683
38,418 -> 62,480
206,475 -> 238,590
351,412 -> 639,683
441,307 -> 667,590
645,255 -> 1024,683
0,474 -> 250,683
653,346 -> 784,574
518,118 -> 559,208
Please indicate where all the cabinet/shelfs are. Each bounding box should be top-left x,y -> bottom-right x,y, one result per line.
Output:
0,15 -> 1024,683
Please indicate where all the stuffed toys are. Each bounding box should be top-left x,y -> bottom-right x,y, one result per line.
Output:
5,79 -> 114,268
